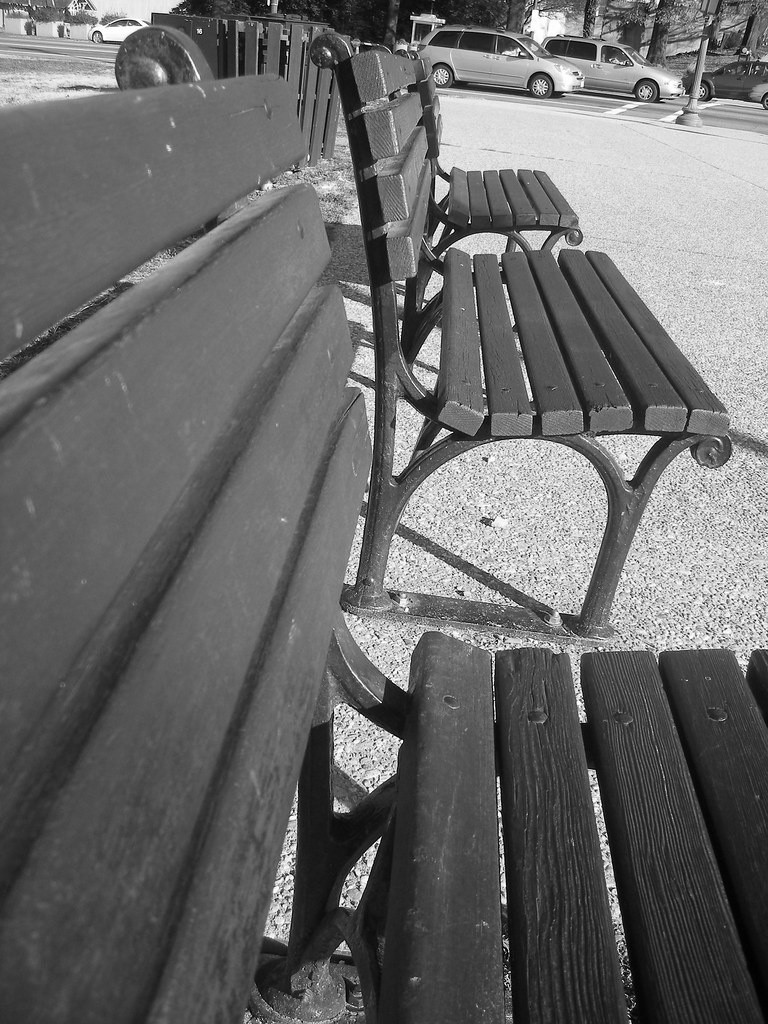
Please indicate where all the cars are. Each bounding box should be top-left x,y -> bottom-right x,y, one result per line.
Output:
87,17 -> 151,45
681,61 -> 768,112
416,24 -> 586,99
538,33 -> 686,103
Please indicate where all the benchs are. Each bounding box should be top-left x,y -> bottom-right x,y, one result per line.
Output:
408,49 -> 584,312
0,29 -> 768,1024
309,33 -> 733,647
